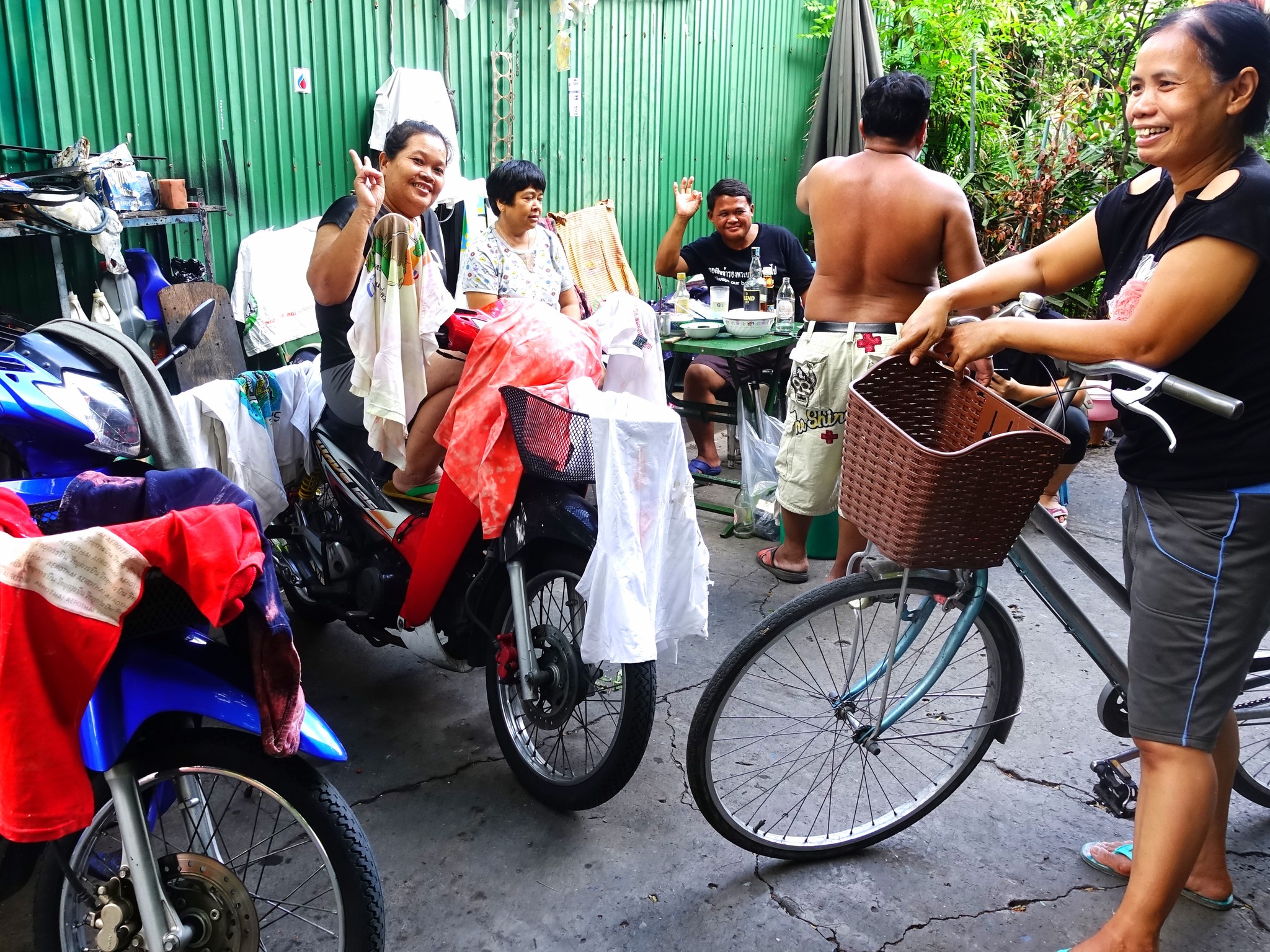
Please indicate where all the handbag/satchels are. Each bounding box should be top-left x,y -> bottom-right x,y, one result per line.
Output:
737,387 -> 784,542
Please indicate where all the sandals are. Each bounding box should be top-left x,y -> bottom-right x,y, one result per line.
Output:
1035,506 -> 1068,531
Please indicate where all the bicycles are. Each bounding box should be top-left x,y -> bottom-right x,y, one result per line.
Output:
689,290 -> 1270,862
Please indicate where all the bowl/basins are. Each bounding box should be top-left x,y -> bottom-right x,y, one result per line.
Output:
669,312 -> 688,317
671,316 -> 694,336
1088,394 -> 1118,421
721,311 -> 778,339
692,312 -> 727,332
679,322 -> 725,339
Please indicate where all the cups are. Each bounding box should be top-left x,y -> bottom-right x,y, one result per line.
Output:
655,312 -> 671,340
710,285 -> 730,312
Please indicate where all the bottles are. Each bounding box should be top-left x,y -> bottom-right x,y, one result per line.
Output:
125,248 -> 172,329
775,277 -> 796,332
763,267 -> 775,313
673,273 -> 690,317
98,260 -> 147,344
137,318 -> 179,396
90,280 -> 123,333
67,282 -> 90,321
744,246 -> 767,312
733,483 -> 754,539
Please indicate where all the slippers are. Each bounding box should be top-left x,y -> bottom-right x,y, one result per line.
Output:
1080,841 -> 1234,911
688,459 -> 722,476
382,480 -> 439,503
756,546 -> 809,581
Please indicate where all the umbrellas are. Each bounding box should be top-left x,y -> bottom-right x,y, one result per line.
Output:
795,0 -> 886,196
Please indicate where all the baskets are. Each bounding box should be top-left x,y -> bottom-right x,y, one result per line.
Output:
0,467 -> 254,643
498,384 -> 680,484
837,355 -> 1070,570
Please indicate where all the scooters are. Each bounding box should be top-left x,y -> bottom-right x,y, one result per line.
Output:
260,314 -> 661,815
0,295 -> 389,952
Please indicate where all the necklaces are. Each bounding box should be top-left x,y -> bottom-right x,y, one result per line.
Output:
496,220 -> 530,251
382,202 -> 419,229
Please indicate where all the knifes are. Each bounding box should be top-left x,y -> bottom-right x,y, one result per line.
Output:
664,334 -> 689,344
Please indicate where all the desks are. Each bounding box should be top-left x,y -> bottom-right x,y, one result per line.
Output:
656,319 -> 804,540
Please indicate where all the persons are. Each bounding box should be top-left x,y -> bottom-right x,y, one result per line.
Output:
939,294 -> 1092,532
755,72 -> 994,581
462,161 -> 581,327
890,0 -> 1270,952
654,177 -> 817,478
307,119 -> 469,504
